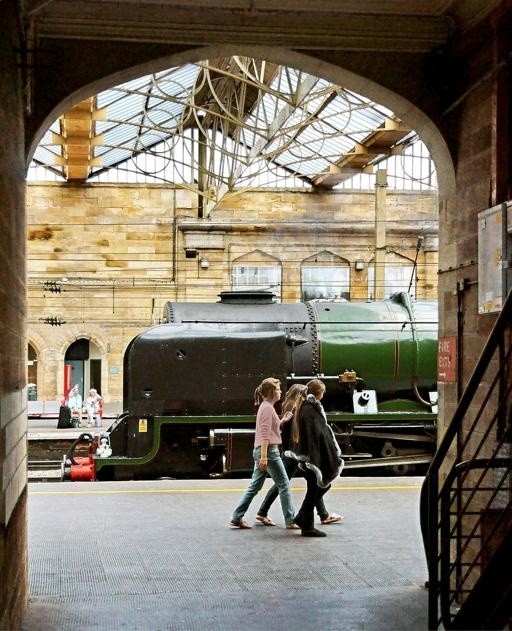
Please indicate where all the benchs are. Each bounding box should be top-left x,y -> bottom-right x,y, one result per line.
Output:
28,399 -> 102,427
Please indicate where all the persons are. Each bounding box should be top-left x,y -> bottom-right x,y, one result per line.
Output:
229,377 -> 300,529
283,379 -> 345,536
83,388 -> 102,427
256,383 -> 342,526
68,384 -> 83,427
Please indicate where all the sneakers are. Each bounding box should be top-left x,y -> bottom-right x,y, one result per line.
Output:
256,514 -> 275,526
293,515 -> 327,537
286,522 -> 300,529
230,519 -> 252,529
321,514 -> 341,525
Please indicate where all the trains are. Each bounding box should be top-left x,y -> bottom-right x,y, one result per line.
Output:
60,291 -> 438,482
